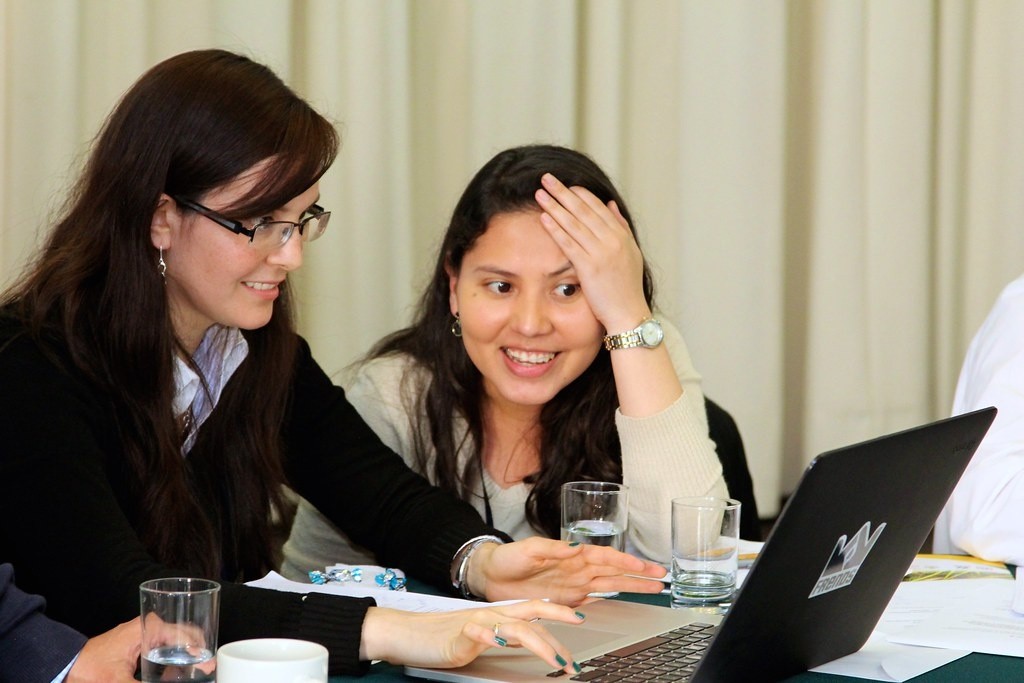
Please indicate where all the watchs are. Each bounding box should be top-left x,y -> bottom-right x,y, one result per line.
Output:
605,316 -> 664,348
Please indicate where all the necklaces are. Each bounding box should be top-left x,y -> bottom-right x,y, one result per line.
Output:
182,408 -> 192,432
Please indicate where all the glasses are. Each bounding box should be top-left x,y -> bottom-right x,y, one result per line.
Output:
170,192 -> 331,251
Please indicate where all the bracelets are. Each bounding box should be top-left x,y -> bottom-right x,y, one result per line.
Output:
451,538 -> 493,599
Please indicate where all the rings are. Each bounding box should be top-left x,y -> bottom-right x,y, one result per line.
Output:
493,623 -> 501,635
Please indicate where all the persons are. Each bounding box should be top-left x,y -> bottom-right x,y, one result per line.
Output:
0,562 -> 216,683
282,144 -> 730,588
0,51 -> 670,683
932,274 -> 1024,562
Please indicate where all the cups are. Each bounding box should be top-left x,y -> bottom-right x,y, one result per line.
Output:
138,577 -> 222,683
215,638 -> 329,683
669,495 -> 743,616
559,480 -> 628,598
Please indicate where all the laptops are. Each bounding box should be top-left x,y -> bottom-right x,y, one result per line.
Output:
405,405 -> 1000,683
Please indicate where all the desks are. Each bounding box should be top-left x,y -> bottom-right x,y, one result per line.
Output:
329,537 -> 1024,683
